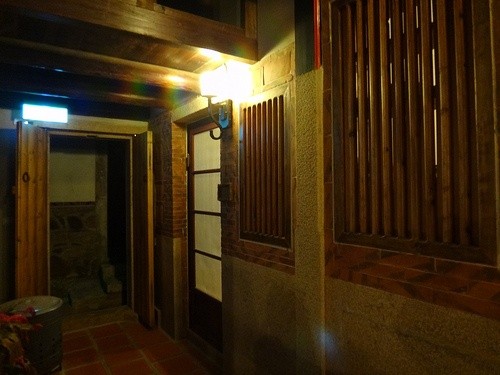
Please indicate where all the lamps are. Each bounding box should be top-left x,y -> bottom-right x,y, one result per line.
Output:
199,69 -> 233,140
